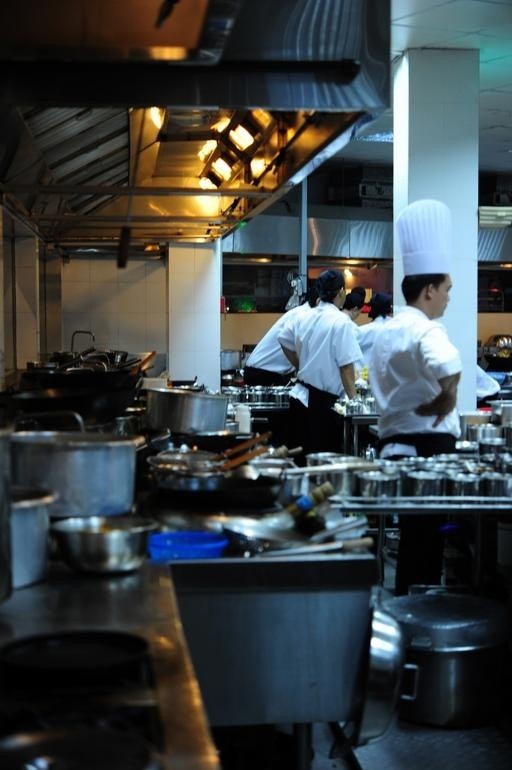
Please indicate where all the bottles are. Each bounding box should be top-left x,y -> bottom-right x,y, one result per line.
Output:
260,483 -> 335,531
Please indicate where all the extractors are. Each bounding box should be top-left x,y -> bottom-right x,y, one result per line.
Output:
17,95 -> 136,218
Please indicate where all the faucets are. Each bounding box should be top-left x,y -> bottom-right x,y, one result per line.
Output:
55,345 -> 112,372
70,329 -> 96,355
17,409 -> 89,433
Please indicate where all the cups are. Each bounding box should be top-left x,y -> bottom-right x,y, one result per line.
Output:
148,530 -> 225,563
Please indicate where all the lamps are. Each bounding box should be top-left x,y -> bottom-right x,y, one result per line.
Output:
196,117 -> 263,193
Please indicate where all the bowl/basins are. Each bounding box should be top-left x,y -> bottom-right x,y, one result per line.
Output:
227,295 -> 257,312
48,515 -> 158,575
305,422 -> 512,496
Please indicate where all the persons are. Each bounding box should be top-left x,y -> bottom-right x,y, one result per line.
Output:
475,366 -> 500,408
276,269 -> 363,466
356,290 -> 393,370
341,293 -> 364,331
243,285 -> 320,447
369,273 -> 461,459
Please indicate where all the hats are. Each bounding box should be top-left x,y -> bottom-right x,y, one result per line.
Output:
316,268 -> 345,296
368,291 -> 392,303
395,199 -> 455,275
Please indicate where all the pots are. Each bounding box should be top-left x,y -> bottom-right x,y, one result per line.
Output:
10,483 -> 61,591
357,615 -> 424,745
266,387 -> 292,407
16,427 -> 171,520
21,347 -> 156,425
145,376 -> 291,495
221,517 -> 367,555
384,584 -> 488,728
